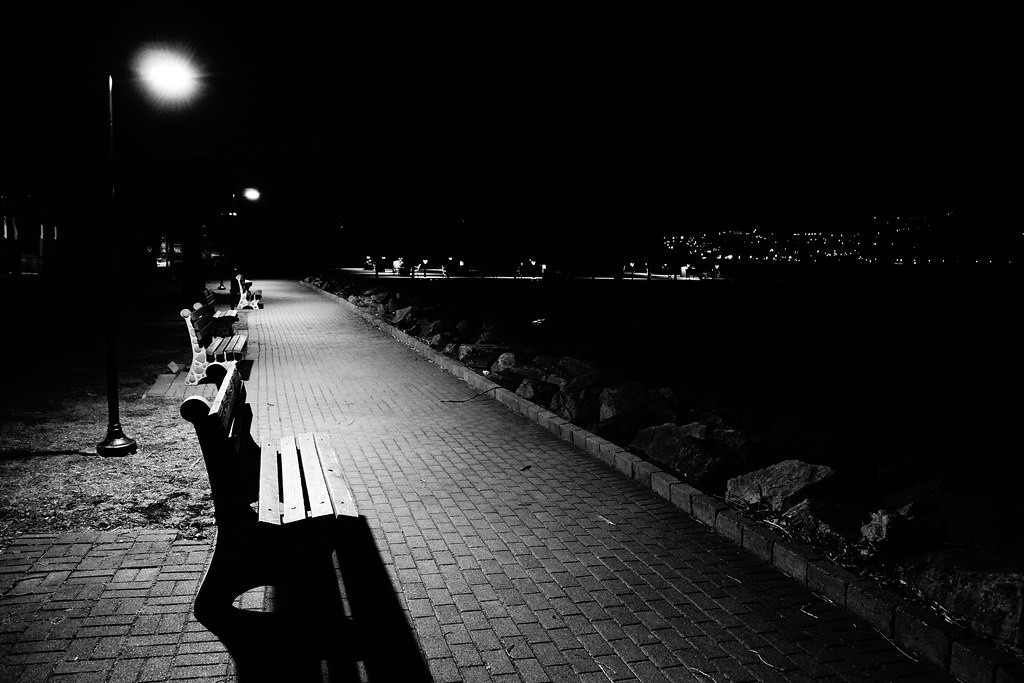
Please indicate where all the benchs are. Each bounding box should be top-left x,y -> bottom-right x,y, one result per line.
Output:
179,302 -> 248,386
235,274 -> 262,309
204,289 -> 239,335
178,362 -> 361,615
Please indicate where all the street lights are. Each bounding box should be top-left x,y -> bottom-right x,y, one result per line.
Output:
95,41 -> 208,458
215,189 -> 260,290
365,255 -> 669,286
